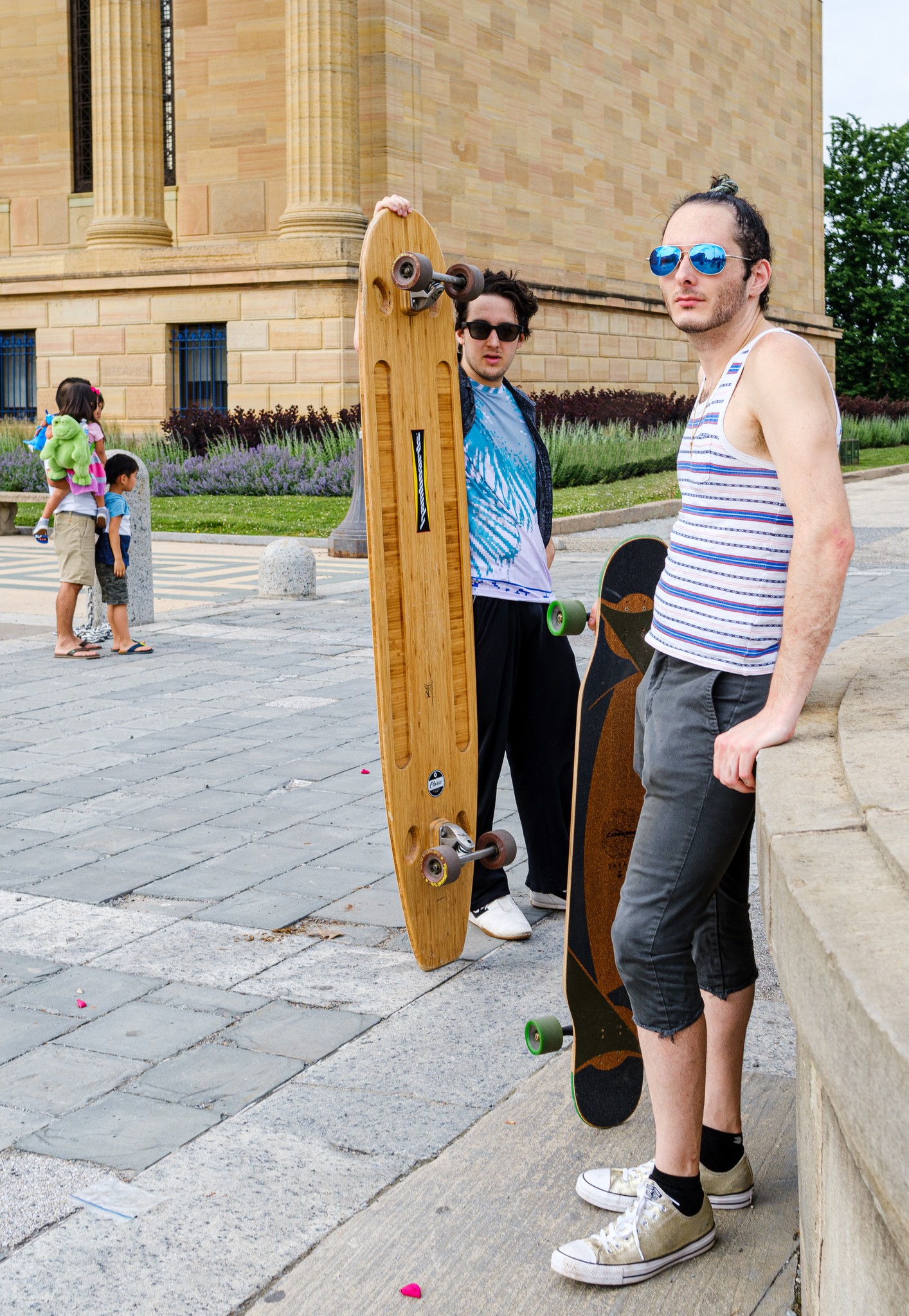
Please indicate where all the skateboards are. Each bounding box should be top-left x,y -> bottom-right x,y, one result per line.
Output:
355,206 -> 479,971
522,532 -> 669,1129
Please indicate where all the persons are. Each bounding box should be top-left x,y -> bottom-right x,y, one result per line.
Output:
94,453 -> 154,654
32,376 -> 108,660
548,177 -> 858,1288
371,191 -> 580,938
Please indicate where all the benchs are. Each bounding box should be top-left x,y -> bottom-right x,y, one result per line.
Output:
0,492 -> 50,535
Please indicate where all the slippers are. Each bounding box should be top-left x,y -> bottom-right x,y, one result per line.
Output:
79,641 -> 102,650
54,647 -> 101,659
111,640 -> 137,652
118,642 -> 154,655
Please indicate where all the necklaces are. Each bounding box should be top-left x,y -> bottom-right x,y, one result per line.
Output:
689,319 -> 768,463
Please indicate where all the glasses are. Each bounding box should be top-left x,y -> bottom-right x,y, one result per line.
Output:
460,320 -> 523,342
646,244 -> 752,275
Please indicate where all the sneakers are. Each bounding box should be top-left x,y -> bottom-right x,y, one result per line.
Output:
576,1153 -> 754,1209
469,894 -> 532,940
550,1178 -> 717,1285
530,888 -> 567,910
